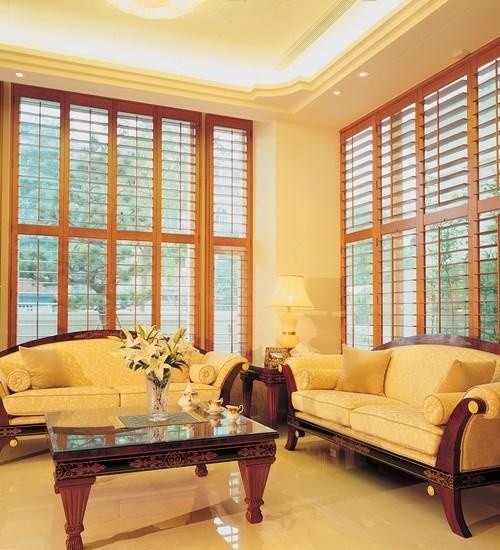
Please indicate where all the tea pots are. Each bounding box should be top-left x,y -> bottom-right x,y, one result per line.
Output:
226,404 -> 243,424
178,390 -> 200,412
228,422 -> 239,435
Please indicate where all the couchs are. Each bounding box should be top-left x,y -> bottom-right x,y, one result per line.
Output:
281,334 -> 500,538
0,330 -> 249,464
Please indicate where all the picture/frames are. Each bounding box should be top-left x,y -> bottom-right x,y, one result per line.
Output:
264,347 -> 292,366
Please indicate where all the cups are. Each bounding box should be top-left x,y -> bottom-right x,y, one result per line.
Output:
207,398 -> 223,412
207,414 -> 222,427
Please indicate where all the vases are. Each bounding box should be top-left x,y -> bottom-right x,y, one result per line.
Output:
147,376 -> 169,421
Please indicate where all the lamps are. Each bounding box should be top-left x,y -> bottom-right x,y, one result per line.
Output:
268,275 -> 318,347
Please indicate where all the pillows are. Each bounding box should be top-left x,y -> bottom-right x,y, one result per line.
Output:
6,346 -> 73,393
423,360 -> 496,426
296,345 -> 392,398
169,364 -> 217,385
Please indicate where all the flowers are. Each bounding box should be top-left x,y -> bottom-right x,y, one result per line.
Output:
107,323 -> 199,411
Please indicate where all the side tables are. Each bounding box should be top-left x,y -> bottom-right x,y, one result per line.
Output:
240,364 -> 286,423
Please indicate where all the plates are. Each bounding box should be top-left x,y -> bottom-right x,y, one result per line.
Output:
204,408 -> 225,417
